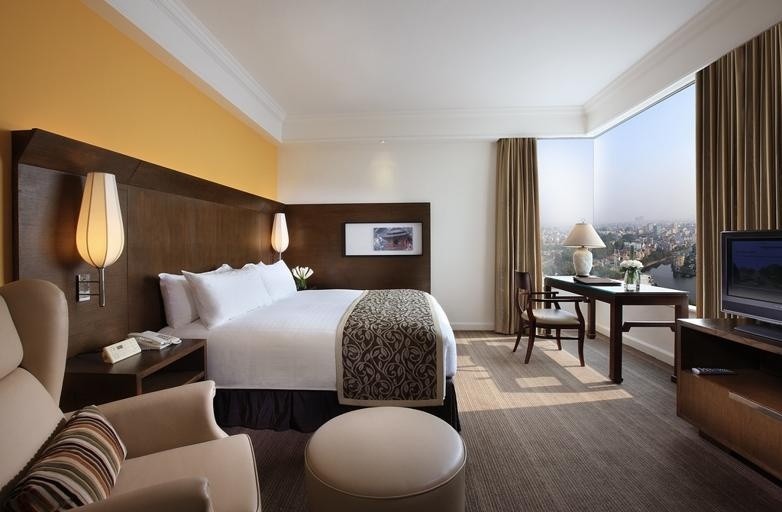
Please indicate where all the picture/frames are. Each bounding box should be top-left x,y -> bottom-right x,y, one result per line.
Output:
342,221 -> 422,258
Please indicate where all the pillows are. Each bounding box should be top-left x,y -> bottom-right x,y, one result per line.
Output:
12,406 -> 127,512
256,260 -> 266,267
258,259 -> 296,298
241,262 -> 276,298
159,264 -> 233,329
180,267 -> 271,328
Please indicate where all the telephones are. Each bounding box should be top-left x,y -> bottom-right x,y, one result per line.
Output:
128,330 -> 182,350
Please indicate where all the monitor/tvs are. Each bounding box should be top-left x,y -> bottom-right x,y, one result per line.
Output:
720,230 -> 782,342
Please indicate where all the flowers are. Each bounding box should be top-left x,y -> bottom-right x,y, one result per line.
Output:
292,266 -> 314,289
619,259 -> 643,283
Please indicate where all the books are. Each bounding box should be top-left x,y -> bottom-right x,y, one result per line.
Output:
573,276 -> 622,287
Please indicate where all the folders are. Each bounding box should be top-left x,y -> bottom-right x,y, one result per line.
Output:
574,277 -> 621,287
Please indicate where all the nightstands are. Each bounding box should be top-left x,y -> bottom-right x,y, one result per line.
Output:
61,335 -> 208,409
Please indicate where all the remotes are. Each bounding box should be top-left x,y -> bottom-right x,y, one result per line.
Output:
692,367 -> 731,375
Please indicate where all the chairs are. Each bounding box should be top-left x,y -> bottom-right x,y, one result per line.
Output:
513,271 -> 591,367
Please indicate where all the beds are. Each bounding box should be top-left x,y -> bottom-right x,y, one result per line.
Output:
157,260 -> 461,432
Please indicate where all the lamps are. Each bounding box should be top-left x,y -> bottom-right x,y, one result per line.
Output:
563,223 -> 606,276
74,172 -> 124,308
271,213 -> 289,260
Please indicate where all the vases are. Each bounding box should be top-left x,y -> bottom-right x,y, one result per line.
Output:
624,270 -> 641,292
299,279 -> 308,289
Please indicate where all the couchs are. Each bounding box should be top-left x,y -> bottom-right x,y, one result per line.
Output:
304,406 -> 465,511
0,279 -> 261,512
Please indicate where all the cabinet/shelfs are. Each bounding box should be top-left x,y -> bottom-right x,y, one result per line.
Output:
676,318 -> 782,482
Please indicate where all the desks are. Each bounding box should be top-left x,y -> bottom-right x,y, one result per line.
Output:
545,276 -> 689,385
